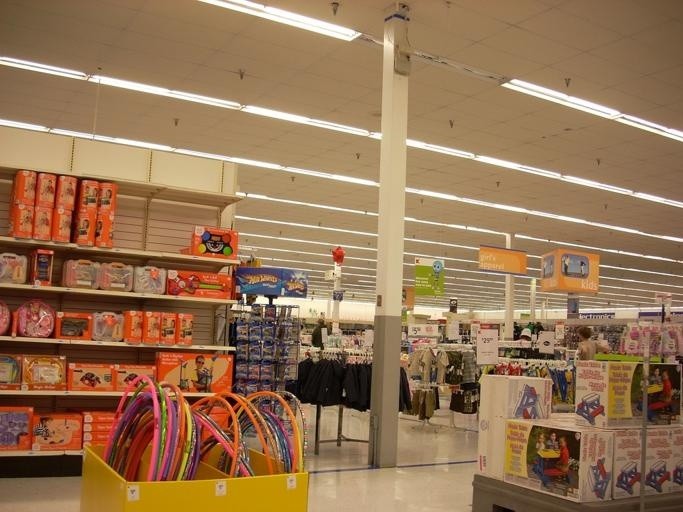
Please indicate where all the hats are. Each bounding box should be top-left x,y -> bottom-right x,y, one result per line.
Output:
520,327 -> 532,338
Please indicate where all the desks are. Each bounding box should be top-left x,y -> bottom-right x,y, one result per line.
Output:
470,474 -> 682,511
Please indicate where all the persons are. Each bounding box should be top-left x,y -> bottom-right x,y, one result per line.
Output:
312,319 -> 330,350
576,259 -> 586,277
514,321 -> 544,342
561,255 -> 571,276
570,298 -> 577,313
573,327 -> 596,361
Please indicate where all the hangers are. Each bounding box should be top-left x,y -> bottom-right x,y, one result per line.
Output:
406,342 -> 475,353
302,347 -> 372,364
497,339 -> 540,351
410,381 -> 463,397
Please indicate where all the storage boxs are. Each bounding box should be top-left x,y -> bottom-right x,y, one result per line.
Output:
166,268 -> 234,299
476,357 -> 681,505
54,310 -> 92,342
0,352 -> 233,454
77,440 -> 308,511
191,224 -> 239,261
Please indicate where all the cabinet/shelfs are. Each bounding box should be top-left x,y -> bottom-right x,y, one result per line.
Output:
0,165 -> 241,454
